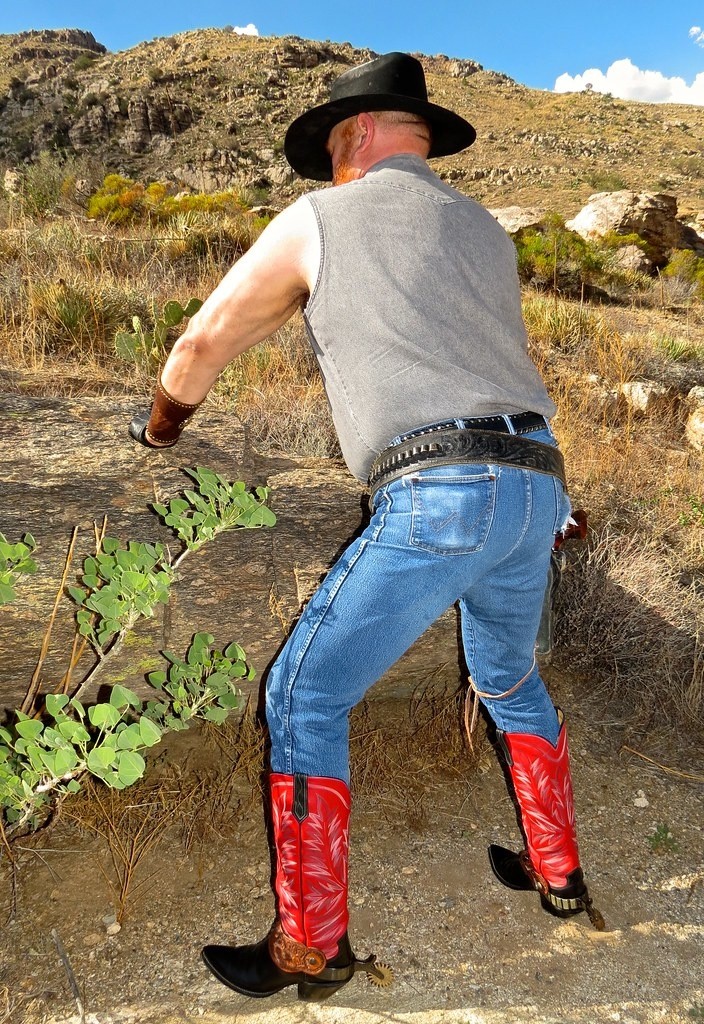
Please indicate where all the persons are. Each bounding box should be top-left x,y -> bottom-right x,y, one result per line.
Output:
128,53 -> 606,1002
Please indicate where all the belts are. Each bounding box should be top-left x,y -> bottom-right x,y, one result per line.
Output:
367,411 -> 567,489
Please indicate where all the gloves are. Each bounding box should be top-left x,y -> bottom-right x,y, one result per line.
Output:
128,407 -> 177,449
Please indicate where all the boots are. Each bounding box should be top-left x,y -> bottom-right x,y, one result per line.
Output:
488,706 -> 606,934
205,771 -> 392,1001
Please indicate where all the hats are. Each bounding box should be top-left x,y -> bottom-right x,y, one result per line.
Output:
283,52 -> 477,181
